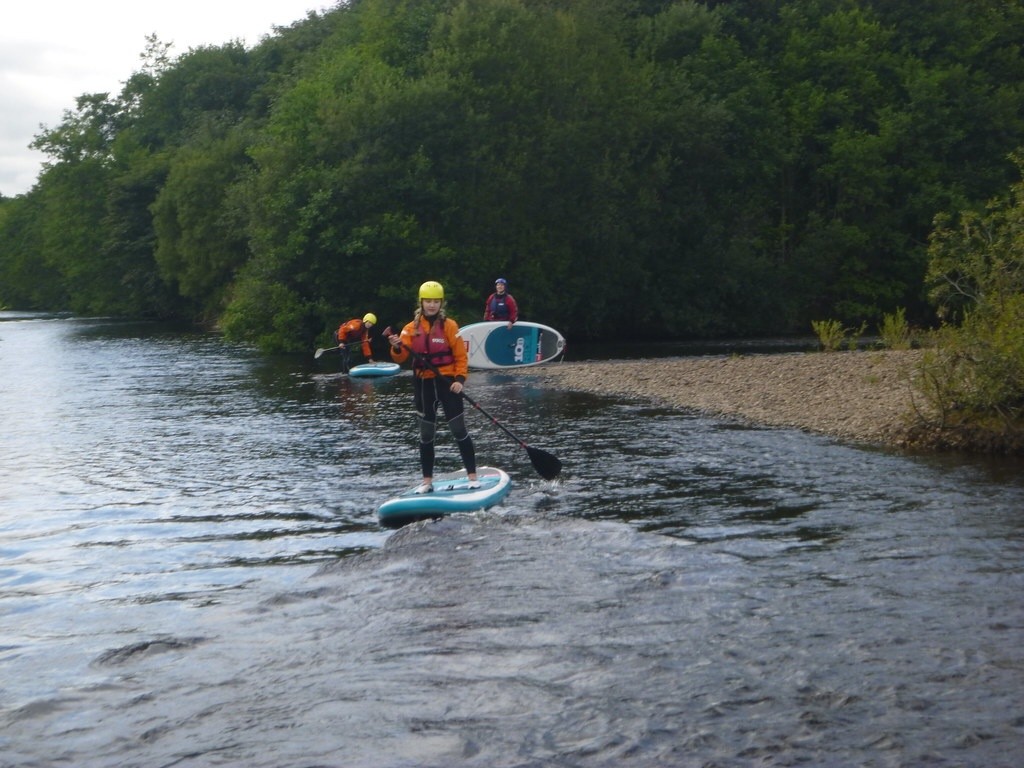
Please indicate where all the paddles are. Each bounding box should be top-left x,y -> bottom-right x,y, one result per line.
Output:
314,337 -> 372,359
382,325 -> 563,482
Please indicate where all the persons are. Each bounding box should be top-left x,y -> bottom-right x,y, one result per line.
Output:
484,278 -> 518,330
334,312 -> 377,375
389,280 -> 481,495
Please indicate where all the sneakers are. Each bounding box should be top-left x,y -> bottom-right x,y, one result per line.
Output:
468,480 -> 481,489
415,484 -> 433,494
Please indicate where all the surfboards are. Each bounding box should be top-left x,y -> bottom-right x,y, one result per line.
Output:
349,361 -> 400,377
378,467 -> 510,524
457,321 -> 566,369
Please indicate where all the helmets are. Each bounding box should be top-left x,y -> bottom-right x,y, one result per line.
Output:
363,312 -> 377,325
419,281 -> 445,302
494,278 -> 507,284
